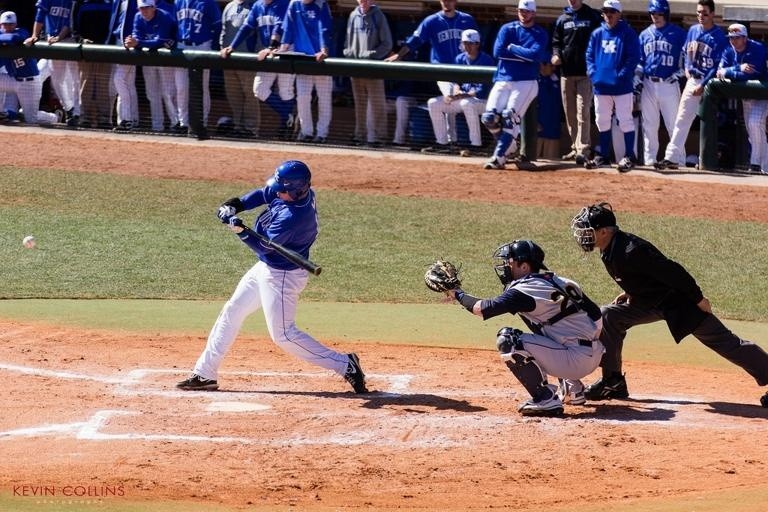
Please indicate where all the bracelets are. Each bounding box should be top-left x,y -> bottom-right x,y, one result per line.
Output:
459,295 -> 480,316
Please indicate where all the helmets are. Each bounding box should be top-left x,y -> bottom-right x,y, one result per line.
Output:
571,202 -> 614,252
490,241 -> 547,281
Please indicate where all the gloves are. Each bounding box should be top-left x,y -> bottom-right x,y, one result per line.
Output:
633,77 -> 644,96
663,71 -> 684,85
229,216 -> 246,235
216,197 -> 245,221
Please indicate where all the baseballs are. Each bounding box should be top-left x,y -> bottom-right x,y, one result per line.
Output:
24,236 -> 36,250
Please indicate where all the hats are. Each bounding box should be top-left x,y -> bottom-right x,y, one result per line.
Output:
0,9 -> 17,27
460,0 -> 748,49
266,162 -> 309,193
135,0 -> 155,9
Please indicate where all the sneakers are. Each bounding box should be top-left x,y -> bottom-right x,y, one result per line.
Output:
176,374 -> 220,391
553,381 -> 584,405
519,395 -> 563,416
52,108 -> 765,173
583,375 -> 630,399
343,352 -> 367,393
759,390 -> 768,407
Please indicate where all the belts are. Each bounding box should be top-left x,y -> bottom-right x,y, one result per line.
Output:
16,78 -> 33,81
694,76 -> 703,79
644,76 -> 660,82
578,339 -> 592,348
180,41 -> 199,46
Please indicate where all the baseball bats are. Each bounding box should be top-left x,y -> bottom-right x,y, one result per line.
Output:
238,221 -> 321,278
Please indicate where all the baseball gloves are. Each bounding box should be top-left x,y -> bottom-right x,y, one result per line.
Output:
423,260 -> 464,295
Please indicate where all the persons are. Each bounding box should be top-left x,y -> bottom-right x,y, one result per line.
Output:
421,240 -> 606,414
176,160 -> 367,394
0,1 -> 767,180
572,202 -> 768,410
